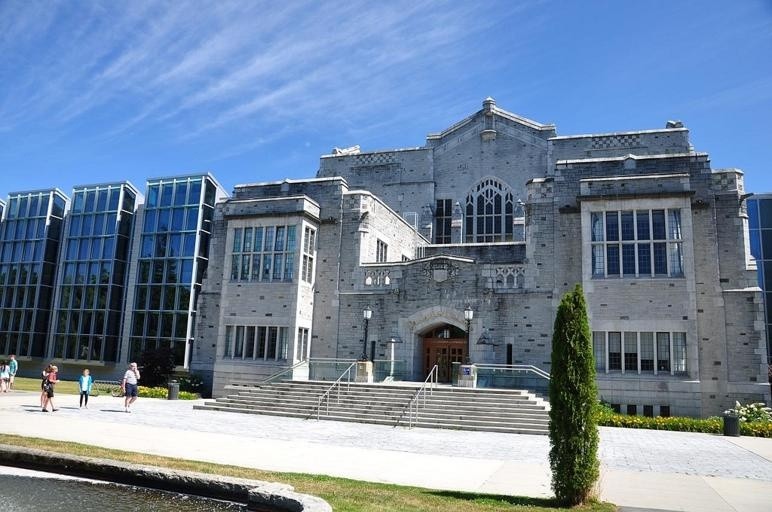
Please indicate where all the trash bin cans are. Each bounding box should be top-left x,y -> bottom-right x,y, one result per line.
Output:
724,414 -> 738,437
451,362 -> 461,386
168,380 -> 181,400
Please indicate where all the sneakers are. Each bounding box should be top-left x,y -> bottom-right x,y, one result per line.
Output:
42,408 -> 58,412
78,406 -> 88,409
125,405 -> 131,413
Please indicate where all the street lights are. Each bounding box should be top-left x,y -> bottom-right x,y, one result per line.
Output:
464,302 -> 475,364
360,303 -> 372,361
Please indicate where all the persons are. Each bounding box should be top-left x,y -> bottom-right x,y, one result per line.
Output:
121,361 -> 141,414
40,363 -> 53,407
0,361 -> 4,394
42,365 -> 60,412
78,368 -> 92,410
8,354 -> 19,384
1,360 -> 14,394
